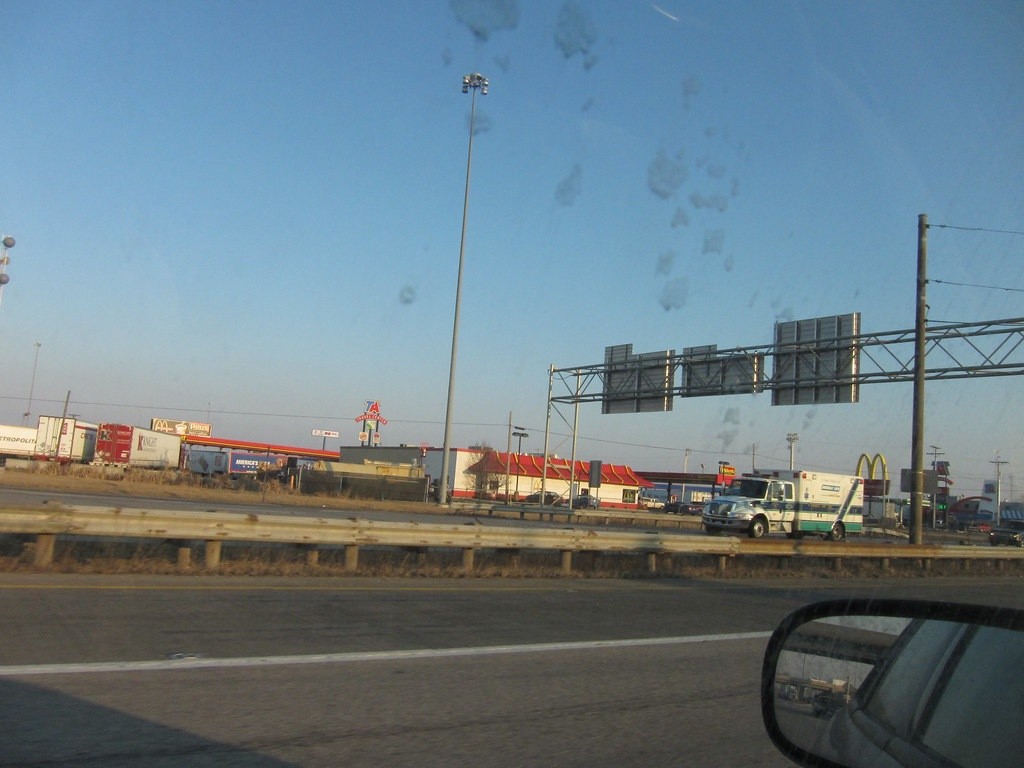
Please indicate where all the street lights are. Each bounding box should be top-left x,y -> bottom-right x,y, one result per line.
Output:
437,72 -> 490,502
513,432 -> 530,502
718,461 -> 730,494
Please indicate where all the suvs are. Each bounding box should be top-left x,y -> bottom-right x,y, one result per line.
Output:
641,496 -> 666,511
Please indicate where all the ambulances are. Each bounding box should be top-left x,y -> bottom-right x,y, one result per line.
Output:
702,469 -> 866,541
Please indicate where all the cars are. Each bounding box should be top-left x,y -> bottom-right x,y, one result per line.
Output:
990,519 -> 1024,547
526,490 -> 564,507
782,683 -> 845,719
577,494 -> 601,510
665,501 -> 708,516
